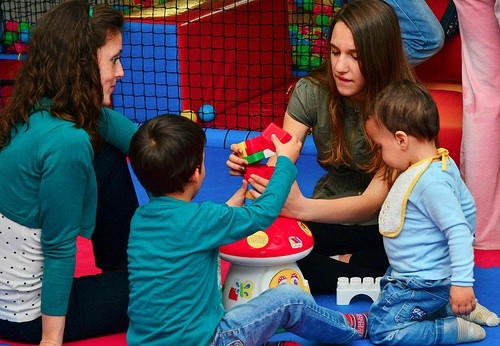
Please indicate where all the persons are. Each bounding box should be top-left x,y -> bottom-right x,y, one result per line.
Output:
126,114 -> 369,346
364,79 -> 500,346
382,0 -> 445,69
226,0 -> 420,294
0,0 -> 139,346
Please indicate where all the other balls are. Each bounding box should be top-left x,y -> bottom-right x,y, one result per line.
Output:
198,104 -> 216,121
0,20 -> 33,53
180,109 -> 197,124
112,0 -> 173,15
